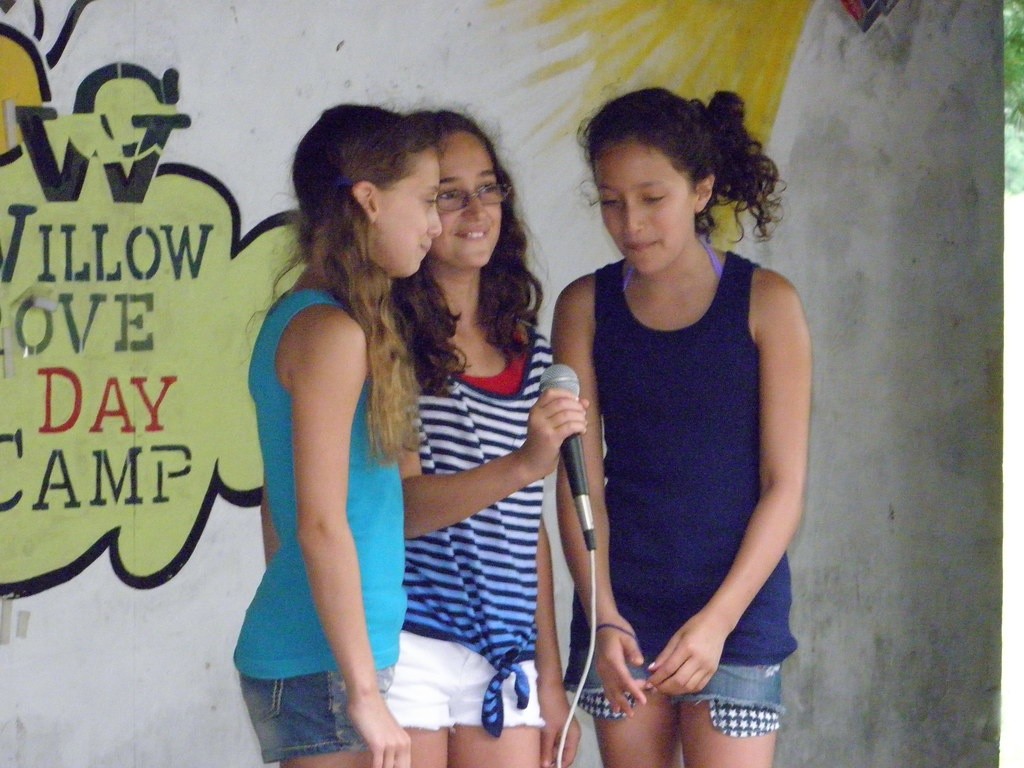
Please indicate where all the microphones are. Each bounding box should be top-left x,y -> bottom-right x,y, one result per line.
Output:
539,364 -> 599,551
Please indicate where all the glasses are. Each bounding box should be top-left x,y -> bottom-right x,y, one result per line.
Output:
436,181 -> 512,211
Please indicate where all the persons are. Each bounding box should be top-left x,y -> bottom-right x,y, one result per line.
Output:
232,100 -> 446,767
542,85 -> 810,767
369,105 -> 592,768
